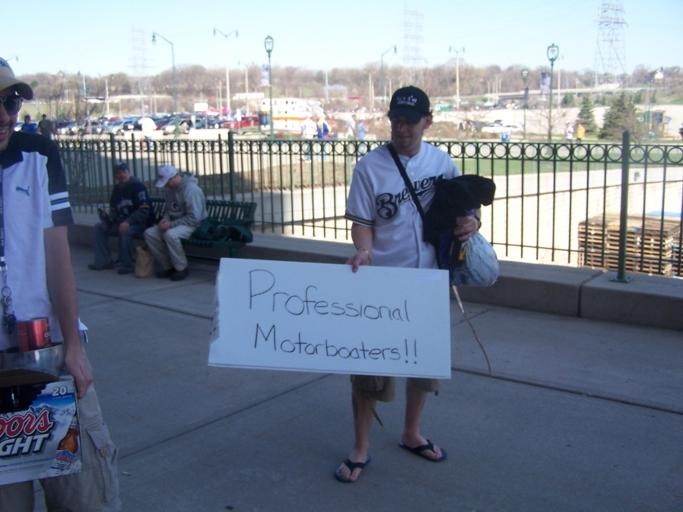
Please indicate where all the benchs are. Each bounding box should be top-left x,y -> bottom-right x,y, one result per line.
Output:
111,198 -> 258,272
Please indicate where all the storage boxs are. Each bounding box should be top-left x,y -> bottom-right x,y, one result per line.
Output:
1,374 -> 82,485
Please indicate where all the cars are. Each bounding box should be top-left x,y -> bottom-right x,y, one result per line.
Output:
328,95 -> 547,134
12,99 -> 322,136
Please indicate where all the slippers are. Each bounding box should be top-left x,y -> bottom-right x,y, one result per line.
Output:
335,455 -> 370,483
398,439 -> 446,462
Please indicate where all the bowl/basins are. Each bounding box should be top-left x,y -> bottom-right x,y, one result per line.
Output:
0,343 -> 65,377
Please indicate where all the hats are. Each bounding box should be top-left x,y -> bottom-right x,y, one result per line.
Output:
389,86 -> 430,123
0,58 -> 33,100
155,165 -> 177,188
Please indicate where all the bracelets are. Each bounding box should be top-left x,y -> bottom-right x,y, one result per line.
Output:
358,249 -> 373,265
472,215 -> 481,231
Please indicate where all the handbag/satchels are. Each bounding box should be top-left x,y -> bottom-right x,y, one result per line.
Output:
453,232 -> 498,289
135,246 -> 155,277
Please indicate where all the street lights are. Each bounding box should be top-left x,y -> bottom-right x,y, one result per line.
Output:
448,45 -> 465,109
263,35 -> 273,140
213,27 -> 239,117
151,31 -> 179,115
545,44 -> 559,143
520,69 -> 530,138
648,70 -> 663,139
380,45 -> 397,102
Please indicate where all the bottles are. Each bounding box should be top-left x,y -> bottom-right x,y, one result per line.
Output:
47,411 -> 77,477
16,316 -> 48,352
97,207 -> 111,225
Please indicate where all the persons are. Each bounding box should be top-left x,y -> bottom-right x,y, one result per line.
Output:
335,85 -> 482,484
21,116 -> 37,134
138,112 -> 158,139
87,163 -> 157,274
143,165 -> 208,281
0,56 -> 122,511
575,123 -> 585,144
300,113 -> 369,162
38,113 -> 60,140
564,122 -> 574,143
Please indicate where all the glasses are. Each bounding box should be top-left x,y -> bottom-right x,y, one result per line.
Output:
2,93 -> 24,115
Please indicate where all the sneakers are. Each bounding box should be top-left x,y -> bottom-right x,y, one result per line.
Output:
118,264 -> 133,273
157,268 -> 189,280
88,262 -> 114,269
